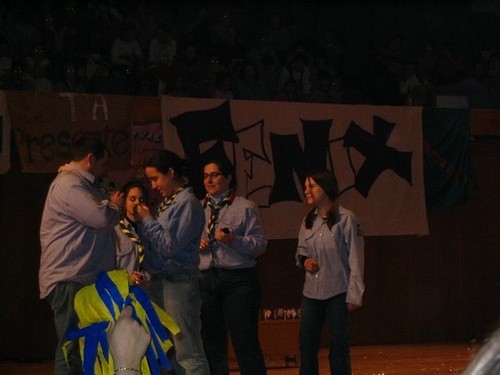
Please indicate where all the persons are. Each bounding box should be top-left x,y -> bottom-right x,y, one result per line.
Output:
296,170 -> 366,375
38,137 -> 268,375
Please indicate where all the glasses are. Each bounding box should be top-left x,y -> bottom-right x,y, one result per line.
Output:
204,172 -> 223,178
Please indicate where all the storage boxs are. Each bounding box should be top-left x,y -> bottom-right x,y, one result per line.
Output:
227,320 -> 301,371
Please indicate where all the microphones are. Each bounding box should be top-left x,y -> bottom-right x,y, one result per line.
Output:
208,228 -> 230,244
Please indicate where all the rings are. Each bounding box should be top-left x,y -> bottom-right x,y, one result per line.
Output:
200,246 -> 202,250
136,281 -> 139,284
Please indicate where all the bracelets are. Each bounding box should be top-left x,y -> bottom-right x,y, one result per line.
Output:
113,367 -> 140,374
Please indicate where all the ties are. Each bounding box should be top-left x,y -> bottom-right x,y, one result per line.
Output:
118,219 -> 144,272
205,190 -> 236,239
155,188 -> 184,219
